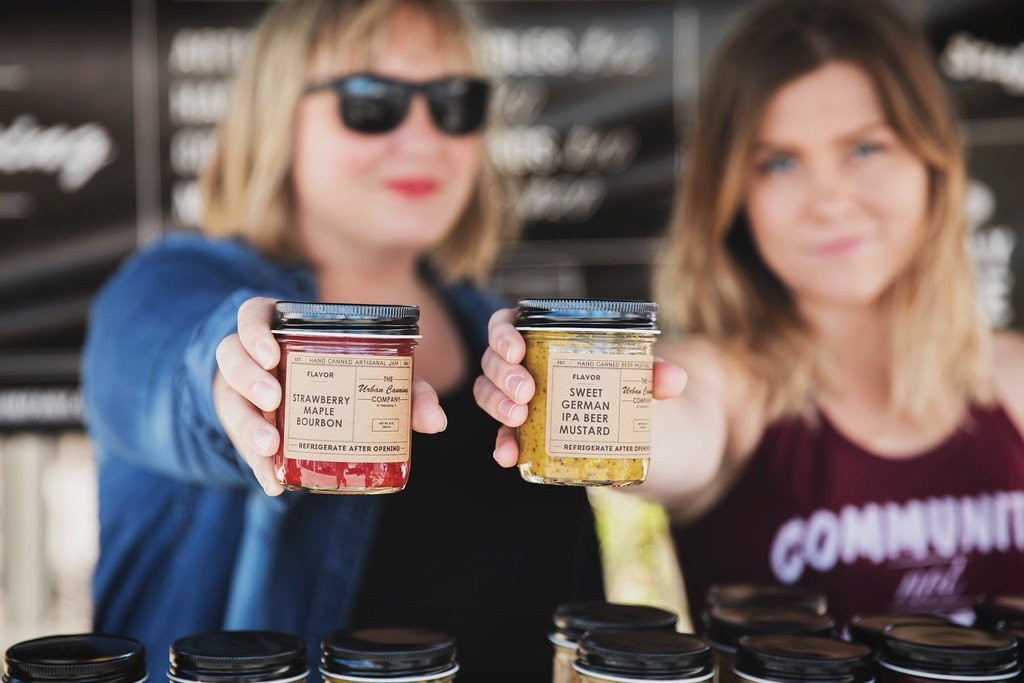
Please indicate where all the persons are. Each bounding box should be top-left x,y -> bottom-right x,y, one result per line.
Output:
473,0 -> 1024,639
80,0 -> 604,683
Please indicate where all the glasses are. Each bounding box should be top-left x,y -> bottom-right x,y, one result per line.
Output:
302,71 -> 490,136
